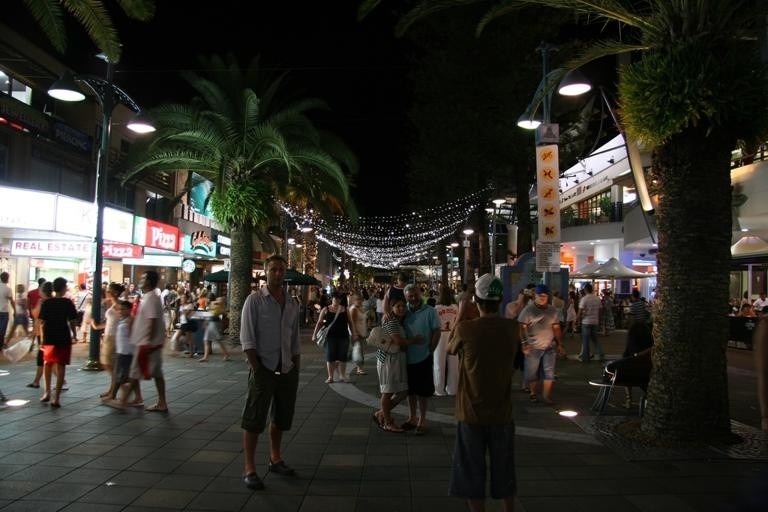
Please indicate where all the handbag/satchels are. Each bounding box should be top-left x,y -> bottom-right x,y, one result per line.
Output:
366,327 -> 400,353
312,304 -> 342,349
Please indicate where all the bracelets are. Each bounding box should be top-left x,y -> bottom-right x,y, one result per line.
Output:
521,338 -> 527,344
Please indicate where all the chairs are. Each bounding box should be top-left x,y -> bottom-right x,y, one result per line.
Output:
594,349 -> 655,417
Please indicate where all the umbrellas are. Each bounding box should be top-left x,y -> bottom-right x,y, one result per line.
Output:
584,257 -> 656,291
565,259 -> 599,282
258,269 -> 320,285
204,270 -> 257,284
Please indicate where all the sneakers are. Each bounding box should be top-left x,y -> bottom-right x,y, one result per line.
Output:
100,392 -> 110,398
101,397 -> 115,401
127,400 -> 144,406
26,383 -> 40,389
222,356 -> 232,361
528,394 -> 538,405
105,400 -> 126,411
39,393 -> 51,402
198,357 -> 210,362
54,385 -> 68,390
541,398 -> 556,405
145,403 -> 168,412
51,402 -> 61,408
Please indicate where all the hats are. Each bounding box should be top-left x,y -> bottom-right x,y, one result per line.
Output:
475,273 -> 503,301
534,284 -> 551,292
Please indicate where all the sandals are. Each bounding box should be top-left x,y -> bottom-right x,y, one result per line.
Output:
267,460 -> 294,475
383,422 -> 397,430
372,410 -> 383,430
400,423 -> 412,431
242,473 -> 263,490
413,426 -> 426,434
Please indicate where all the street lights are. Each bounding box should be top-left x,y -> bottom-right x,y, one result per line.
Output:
46,42 -> 157,372
284,220 -> 314,270
450,215 -> 475,284
515,39 -> 594,285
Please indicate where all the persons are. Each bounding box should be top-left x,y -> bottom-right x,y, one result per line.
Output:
446,273 -> 527,511
26,278 -> 45,345
37,278 -> 75,408
433,286 -> 461,396
552,291 -> 579,337
163,280 -> 235,364
0,273 -> 16,354
103,302 -> 144,406
522,281 -> 535,309
621,312 -> 653,409
240,255 -> 301,488
372,297 -> 425,432
26,282 -> 68,390
311,293 -> 356,384
102,282 -> 126,400
100,271 -> 169,412
625,289 -> 651,354
402,285 -> 440,436
506,291 -> 530,395
73,283 -> 105,344
6,285 -> 29,349
731,292 -> 767,324
601,288 -> 630,334
119,280 -> 143,300
518,285 -> 562,403
318,276 -> 443,302
351,294 -> 369,374
573,284 -> 605,364
458,278 -> 482,323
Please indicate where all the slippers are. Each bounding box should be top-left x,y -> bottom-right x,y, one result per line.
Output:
355,370 -> 367,375
339,377 -> 351,383
323,378 -> 334,385
